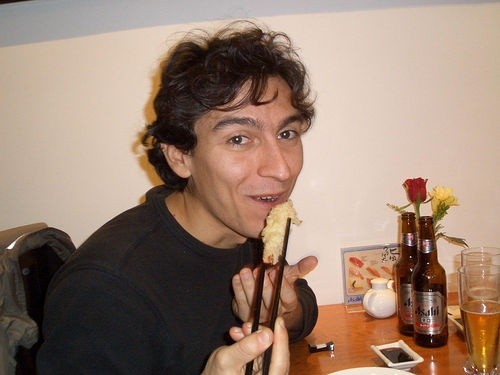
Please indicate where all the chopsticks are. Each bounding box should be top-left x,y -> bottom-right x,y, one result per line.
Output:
245,218 -> 291,375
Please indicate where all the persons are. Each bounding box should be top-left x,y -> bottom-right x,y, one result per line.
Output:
33,18 -> 318,375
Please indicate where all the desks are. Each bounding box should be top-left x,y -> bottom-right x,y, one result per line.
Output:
290,292 -> 468,375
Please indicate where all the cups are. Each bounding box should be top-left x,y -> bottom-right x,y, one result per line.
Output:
458,263 -> 500,375
461,247 -> 500,267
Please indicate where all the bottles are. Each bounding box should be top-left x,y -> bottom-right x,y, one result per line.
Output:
394,212 -> 418,337
411,216 -> 448,348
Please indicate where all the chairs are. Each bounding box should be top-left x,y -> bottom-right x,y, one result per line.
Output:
0,221 -> 75,374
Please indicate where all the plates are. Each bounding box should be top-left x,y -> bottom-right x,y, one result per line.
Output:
370,339 -> 424,371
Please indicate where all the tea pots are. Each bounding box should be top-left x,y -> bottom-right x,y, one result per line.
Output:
363,277 -> 396,318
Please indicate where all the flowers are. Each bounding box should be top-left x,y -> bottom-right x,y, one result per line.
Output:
386,176 -> 469,249
